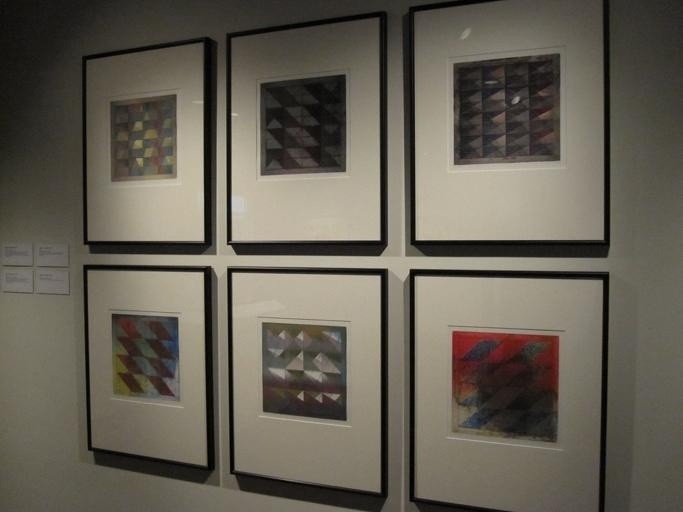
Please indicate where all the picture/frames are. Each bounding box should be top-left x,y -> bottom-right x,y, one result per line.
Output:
224,265 -> 390,501
80,34 -> 220,248
80,263 -> 214,476
402,3 -> 615,247
223,7 -> 391,248
407,267 -> 612,512
